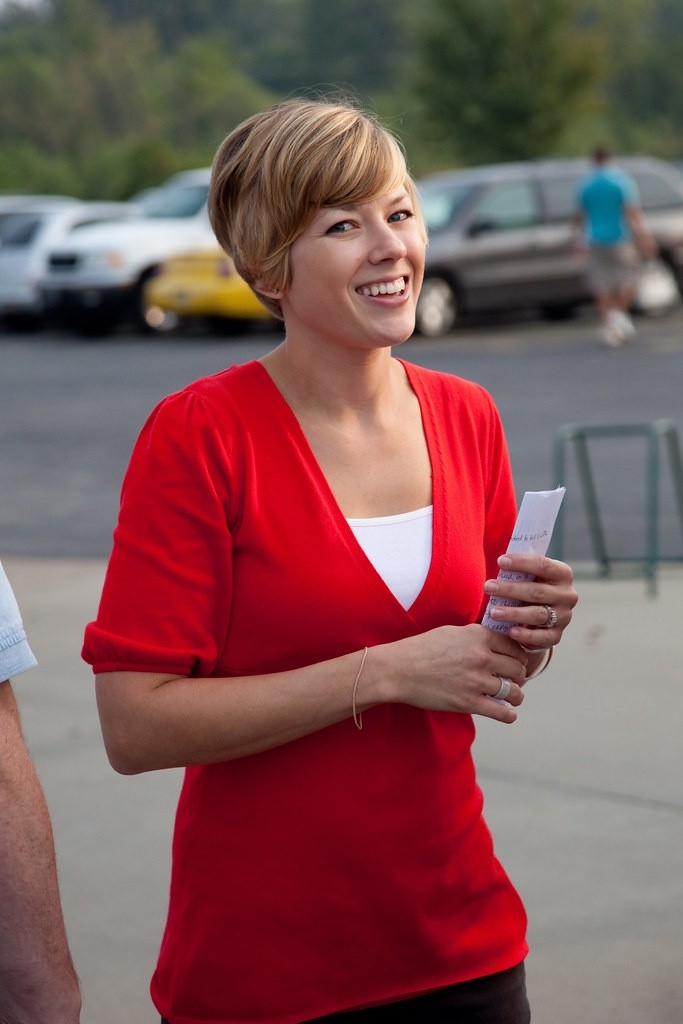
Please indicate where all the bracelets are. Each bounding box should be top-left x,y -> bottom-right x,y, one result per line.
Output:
353,646 -> 368,729
519,643 -> 547,654
524,646 -> 554,681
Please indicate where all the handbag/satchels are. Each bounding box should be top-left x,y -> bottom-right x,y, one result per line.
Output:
634,259 -> 679,317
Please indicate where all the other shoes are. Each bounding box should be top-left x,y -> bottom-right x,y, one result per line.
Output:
597,306 -> 636,350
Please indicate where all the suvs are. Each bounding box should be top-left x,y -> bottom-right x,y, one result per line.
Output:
41,165 -> 230,335
400,150 -> 683,340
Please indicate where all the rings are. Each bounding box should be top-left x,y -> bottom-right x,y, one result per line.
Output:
493,675 -> 512,700
538,603 -> 559,628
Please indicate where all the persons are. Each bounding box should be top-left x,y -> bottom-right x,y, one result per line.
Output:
0,567 -> 84,1024
572,140 -> 655,348
79,95 -> 578,1024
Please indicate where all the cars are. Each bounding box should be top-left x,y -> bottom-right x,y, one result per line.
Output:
140,231 -> 285,340
0,194 -> 130,331
